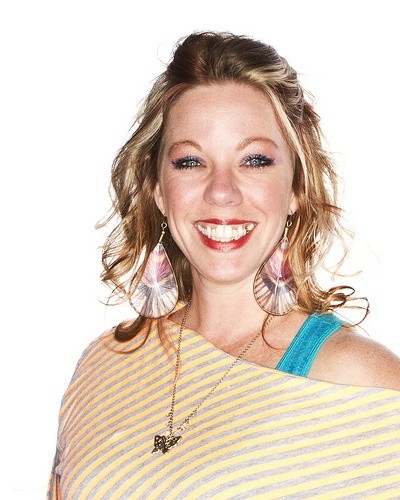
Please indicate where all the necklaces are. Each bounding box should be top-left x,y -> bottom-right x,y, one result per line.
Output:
151,295 -> 279,454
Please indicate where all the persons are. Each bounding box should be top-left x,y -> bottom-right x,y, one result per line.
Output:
46,31 -> 399,500
